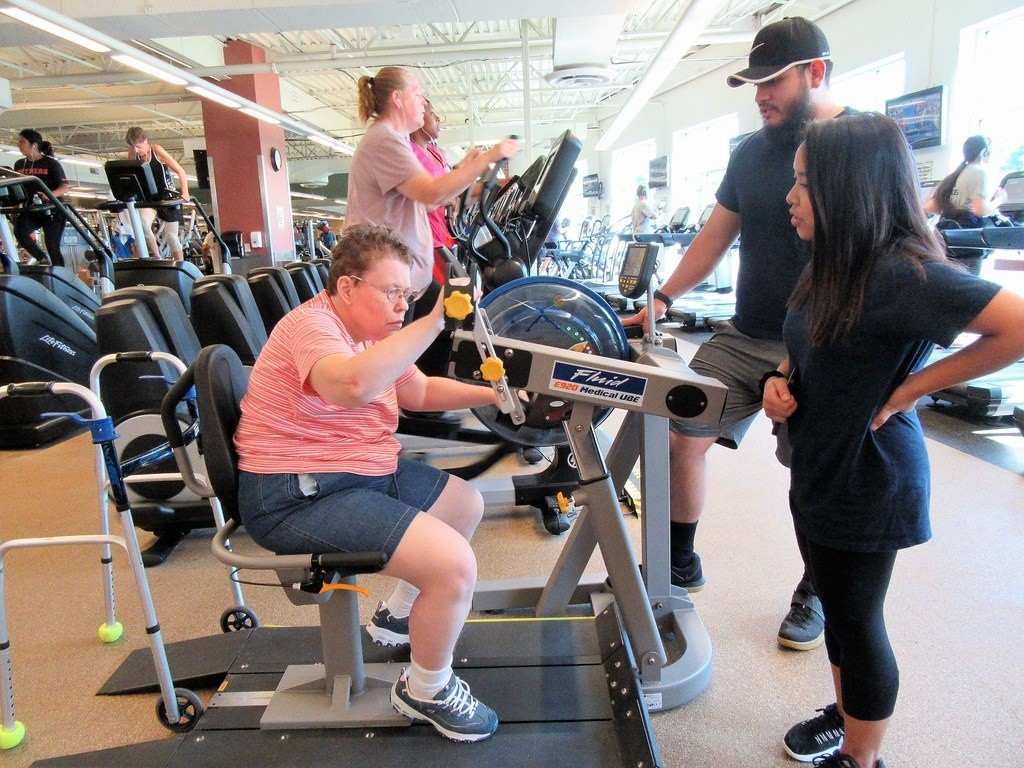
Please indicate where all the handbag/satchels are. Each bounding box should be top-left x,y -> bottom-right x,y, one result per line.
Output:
936,208 -> 985,259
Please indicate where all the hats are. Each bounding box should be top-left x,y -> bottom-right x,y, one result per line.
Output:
727,16 -> 831,88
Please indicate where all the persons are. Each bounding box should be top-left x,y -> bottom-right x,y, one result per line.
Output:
84,250 -> 100,274
539,214 -> 573,275
14,128 -> 69,267
233,222 -> 529,742
621,17 -> 864,650
340,67 -> 517,287
762,110 -> 1024,768
923,133 -> 1009,277
96,215 -> 221,275
632,184 -> 665,285
126,126 -> 189,261
294,218 -> 337,255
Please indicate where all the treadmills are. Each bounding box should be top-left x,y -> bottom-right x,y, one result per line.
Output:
570,203 -> 1023,432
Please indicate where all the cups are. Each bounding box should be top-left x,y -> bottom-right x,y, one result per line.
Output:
445,144 -> 482,183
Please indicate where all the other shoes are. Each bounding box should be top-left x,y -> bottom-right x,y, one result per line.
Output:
408,409 -> 462,427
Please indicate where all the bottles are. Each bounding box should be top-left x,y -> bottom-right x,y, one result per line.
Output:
465,223 -> 493,249
33,194 -> 42,204
93,272 -> 101,298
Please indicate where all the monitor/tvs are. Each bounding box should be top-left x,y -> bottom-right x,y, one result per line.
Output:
105,159 -> 158,201
669,208 -> 692,228
697,204 -> 716,226
884,85 -> 943,149
583,174 -> 599,198
483,129 -> 582,225
649,155 -> 669,189
0,165 -> 28,207
730,132 -> 755,154
990,170 -> 1024,215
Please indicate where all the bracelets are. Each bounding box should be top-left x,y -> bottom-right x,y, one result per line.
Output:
653,289 -> 673,308
758,369 -> 785,395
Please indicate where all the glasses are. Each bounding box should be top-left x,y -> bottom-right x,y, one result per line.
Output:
349,273 -> 419,304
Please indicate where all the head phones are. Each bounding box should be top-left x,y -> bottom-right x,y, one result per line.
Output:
642,186 -> 646,195
977,135 -> 990,159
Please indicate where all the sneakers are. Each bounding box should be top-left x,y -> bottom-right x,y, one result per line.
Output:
365,601 -> 410,648
606,553 -> 707,595
783,704 -> 845,762
390,664 -> 499,743
810,749 -> 885,768
778,576 -> 827,649
